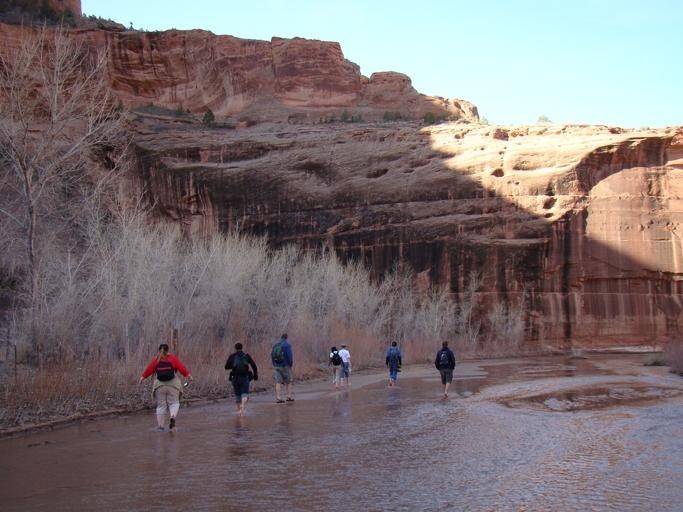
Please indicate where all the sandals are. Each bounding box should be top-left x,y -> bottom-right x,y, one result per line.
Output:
286,397 -> 294,401
276,398 -> 285,403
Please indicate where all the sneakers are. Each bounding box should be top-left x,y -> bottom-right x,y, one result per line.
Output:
169,418 -> 175,429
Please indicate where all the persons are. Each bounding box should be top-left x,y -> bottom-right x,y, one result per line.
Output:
267,403 -> 290,456
386,387 -> 400,422
341,386 -> 349,418
385,341 -> 401,387
227,416 -> 255,470
270,332 -> 295,404
435,339 -> 455,399
328,344 -> 345,389
338,343 -> 352,388
224,342 -> 258,417
135,342 -> 194,433
331,387 -> 343,422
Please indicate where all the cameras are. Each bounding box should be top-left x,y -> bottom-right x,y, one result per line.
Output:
183,381 -> 189,387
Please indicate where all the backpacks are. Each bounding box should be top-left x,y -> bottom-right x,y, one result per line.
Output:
332,351 -> 342,365
155,361 -> 175,382
232,353 -> 250,376
390,349 -> 398,363
439,350 -> 450,368
271,341 -> 284,365
